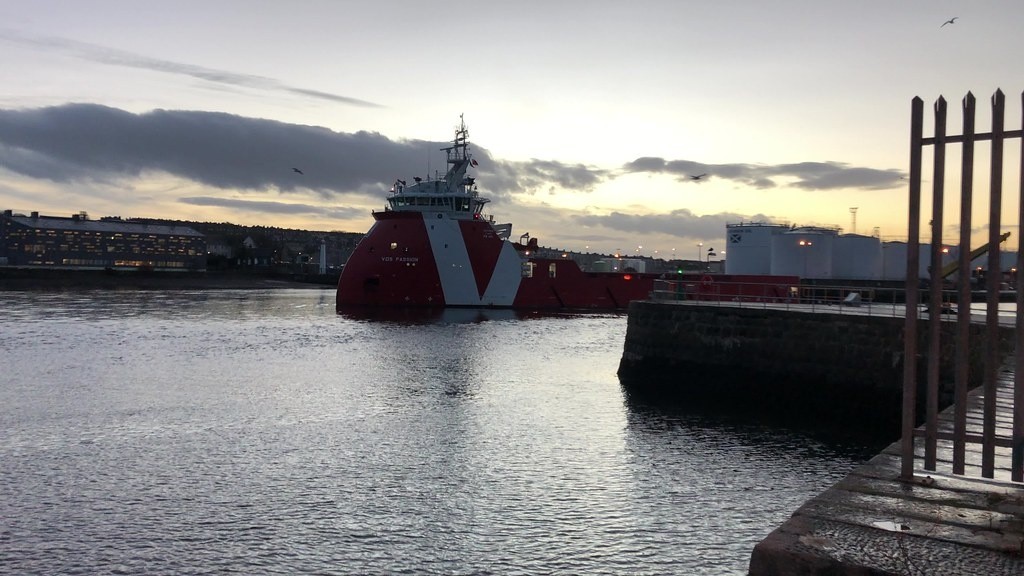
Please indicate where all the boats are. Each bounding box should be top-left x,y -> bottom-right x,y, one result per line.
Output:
337,114 -> 803,309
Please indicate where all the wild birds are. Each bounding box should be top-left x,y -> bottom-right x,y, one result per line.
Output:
940,17 -> 958,28
292,167 -> 303,175
691,174 -> 705,180
